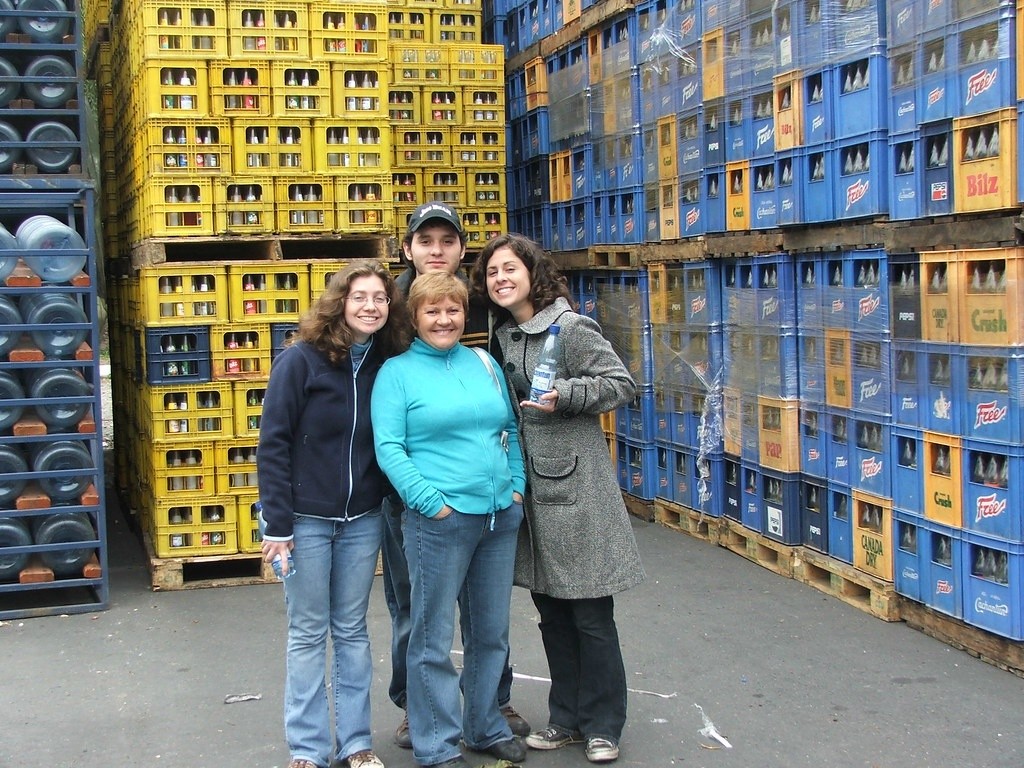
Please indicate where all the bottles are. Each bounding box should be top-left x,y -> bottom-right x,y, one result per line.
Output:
0,295 -> 24,357
0,122 -> 22,174
0,0 -> 16,44
19,292 -> 88,357
22,54 -> 75,105
24,366 -> 92,427
0,516 -> 32,582
0,222 -> 18,284
30,502 -> 96,571
0,56 -> 20,107
490,0 -> 1009,585
121,12 -> 510,248
0,444 -> 28,505
25,122 -> 78,173
17,0 -> 69,44
150,271 -> 303,554
29,441 -> 94,500
17,215 -> 87,282
0,370 -> 24,434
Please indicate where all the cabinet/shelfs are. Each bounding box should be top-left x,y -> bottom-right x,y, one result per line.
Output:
0,0 -> 88,179
0,179 -> 111,621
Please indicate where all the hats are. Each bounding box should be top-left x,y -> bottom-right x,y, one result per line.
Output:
408,201 -> 463,234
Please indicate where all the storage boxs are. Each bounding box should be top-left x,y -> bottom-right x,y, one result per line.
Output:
84,0 -> 1024,642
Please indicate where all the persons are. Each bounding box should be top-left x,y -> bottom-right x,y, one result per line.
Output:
469,232 -> 645,762
257,259 -> 414,768
370,202 -> 531,768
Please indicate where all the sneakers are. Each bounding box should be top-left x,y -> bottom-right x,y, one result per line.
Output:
499,705 -> 531,736
288,759 -> 316,768
526,727 -> 587,749
346,750 -> 385,768
585,737 -> 619,761
395,711 -> 413,747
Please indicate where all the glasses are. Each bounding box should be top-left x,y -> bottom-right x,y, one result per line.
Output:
345,292 -> 391,306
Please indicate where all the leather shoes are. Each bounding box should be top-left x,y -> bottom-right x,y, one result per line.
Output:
487,738 -> 527,762
427,755 -> 471,768
